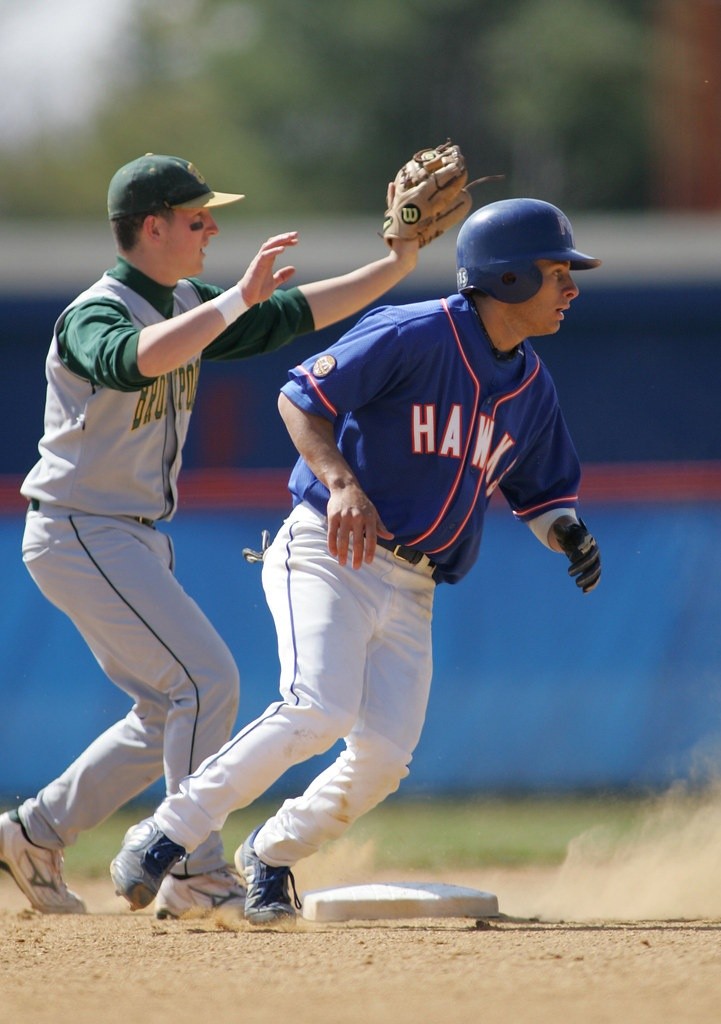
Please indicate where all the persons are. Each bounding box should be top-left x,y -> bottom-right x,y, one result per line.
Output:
0,144 -> 474,918
112,196 -> 602,924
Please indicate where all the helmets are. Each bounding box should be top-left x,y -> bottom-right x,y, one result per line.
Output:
456,199 -> 603,304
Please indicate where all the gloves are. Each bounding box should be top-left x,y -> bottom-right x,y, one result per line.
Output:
551,518 -> 602,593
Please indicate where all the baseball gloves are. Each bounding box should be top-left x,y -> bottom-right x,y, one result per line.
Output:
383,139 -> 476,249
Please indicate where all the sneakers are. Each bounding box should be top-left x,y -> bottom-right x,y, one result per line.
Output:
155,863 -> 248,920
109,815 -> 187,912
0,808 -> 87,914
233,824 -> 302,923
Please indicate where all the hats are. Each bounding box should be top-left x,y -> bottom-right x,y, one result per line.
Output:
108,153 -> 245,220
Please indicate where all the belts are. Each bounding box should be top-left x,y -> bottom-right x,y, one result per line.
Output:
363,531 -> 445,585
31,498 -> 154,527
